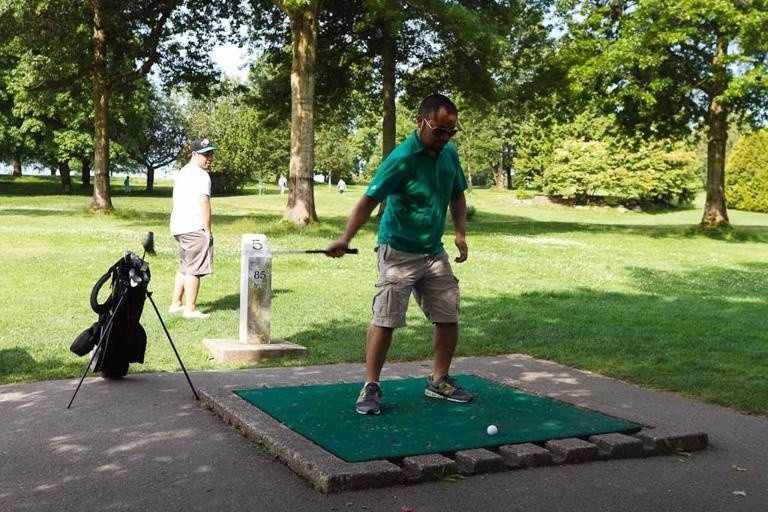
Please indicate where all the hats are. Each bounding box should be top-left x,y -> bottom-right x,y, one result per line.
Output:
190,139 -> 219,153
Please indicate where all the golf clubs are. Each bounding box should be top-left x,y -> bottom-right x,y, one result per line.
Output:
122,251 -> 149,289
232,249 -> 358,254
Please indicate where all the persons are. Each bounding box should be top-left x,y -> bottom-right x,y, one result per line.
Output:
327,94 -> 475,415
336,177 -> 346,195
123,176 -> 131,194
258,178 -> 265,196
168,138 -> 217,319
278,173 -> 288,194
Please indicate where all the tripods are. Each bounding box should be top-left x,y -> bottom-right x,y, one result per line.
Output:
67,284 -> 199,409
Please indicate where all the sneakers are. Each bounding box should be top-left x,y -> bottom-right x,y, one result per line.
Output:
183,310 -> 209,319
425,375 -> 473,402
169,305 -> 184,312
356,383 -> 382,414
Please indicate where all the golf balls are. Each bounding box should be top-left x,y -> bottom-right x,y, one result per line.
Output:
487,424 -> 498,436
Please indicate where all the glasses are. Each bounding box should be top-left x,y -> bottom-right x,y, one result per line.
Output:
424,119 -> 457,137
